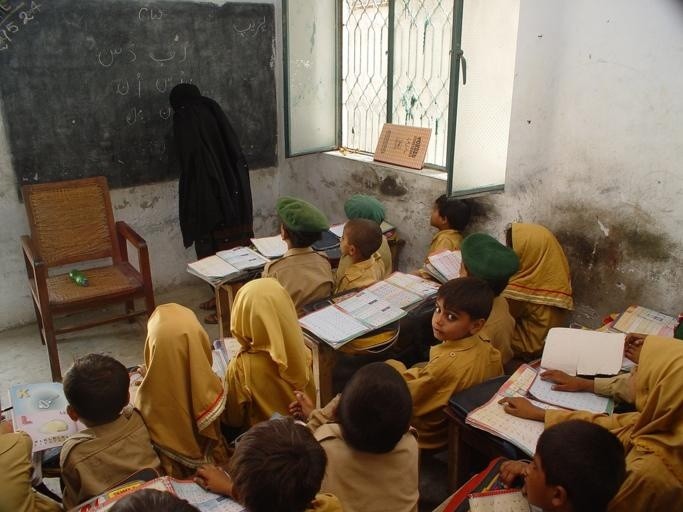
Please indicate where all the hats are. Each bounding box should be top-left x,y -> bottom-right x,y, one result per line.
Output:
460,232 -> 519,280
344,194 -> 384,224
277,197 -> 329,233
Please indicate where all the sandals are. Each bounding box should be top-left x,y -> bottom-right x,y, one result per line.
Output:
199,297 -> 216,309
205,312 -> 218,324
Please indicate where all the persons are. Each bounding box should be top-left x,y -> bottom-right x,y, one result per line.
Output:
168,82 -> 254,325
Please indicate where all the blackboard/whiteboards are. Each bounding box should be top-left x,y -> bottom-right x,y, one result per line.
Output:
0,0 -> 279,203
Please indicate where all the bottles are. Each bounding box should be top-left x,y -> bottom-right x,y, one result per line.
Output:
68,269 -> 89,287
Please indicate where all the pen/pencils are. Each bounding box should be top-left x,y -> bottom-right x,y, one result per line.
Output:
0,406 -> 13,414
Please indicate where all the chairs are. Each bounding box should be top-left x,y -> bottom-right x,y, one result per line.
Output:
19,175 -> 152,381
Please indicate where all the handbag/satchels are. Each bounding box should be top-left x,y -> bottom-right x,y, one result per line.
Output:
337,320 -> 402,356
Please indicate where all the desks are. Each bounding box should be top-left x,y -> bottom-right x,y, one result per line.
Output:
440,321 -> 619,502
216,238 -> 403,339
295,271 -> 437,409
